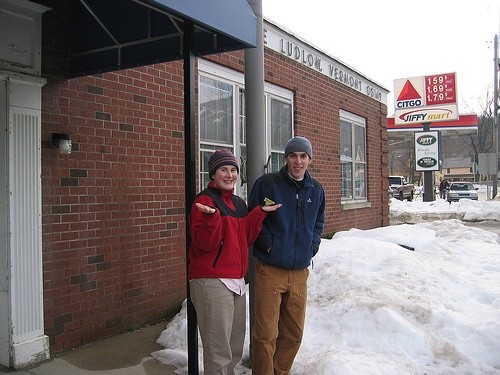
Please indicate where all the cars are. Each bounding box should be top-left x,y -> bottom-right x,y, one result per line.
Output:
441,181 -> 479,202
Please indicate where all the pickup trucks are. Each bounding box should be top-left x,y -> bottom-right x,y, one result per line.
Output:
388,175 -> 415,200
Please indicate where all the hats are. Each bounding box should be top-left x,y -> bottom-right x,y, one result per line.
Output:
209,150 -> 239,181
284,136 -> 312,159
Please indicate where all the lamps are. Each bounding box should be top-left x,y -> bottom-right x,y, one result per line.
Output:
51,132 -> 71,155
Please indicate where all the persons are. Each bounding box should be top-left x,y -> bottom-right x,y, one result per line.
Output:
248,136 -> 325,375
440,180 -> 445,199
187,149 -> 282,375
443,179 -> 449,199
